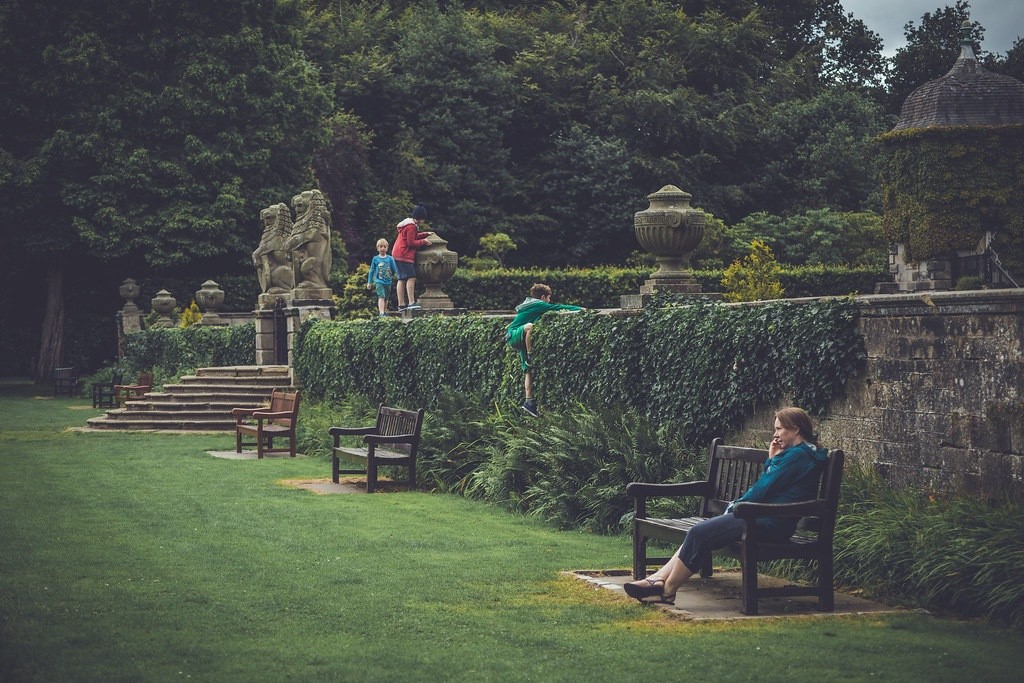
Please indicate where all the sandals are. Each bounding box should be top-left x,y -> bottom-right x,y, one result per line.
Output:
637,594 -> 676,605
624,579 -> 665,598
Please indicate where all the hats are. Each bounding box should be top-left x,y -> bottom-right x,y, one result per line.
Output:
412,202 -> 427,219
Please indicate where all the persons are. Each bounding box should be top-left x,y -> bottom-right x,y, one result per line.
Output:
624,407 -> 829,605
392,202 -> 434,312
367,239 -> 396,317
507,284 -> 588,418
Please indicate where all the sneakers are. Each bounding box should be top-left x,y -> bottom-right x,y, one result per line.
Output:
407,302 -> 421,309
521,402 -> 538,418
398,304 -> 408,312
525,359 -> 532,367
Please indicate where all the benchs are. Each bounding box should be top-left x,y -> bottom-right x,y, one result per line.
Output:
114,373 -> 153,402
329,404 -> 424,493
92,374 -> 122,409
232,388 -> 301,458
55,368 -> 80,396
626,438 -> 845,615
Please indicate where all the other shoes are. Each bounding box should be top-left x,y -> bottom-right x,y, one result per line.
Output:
379,313 -> 389,317
